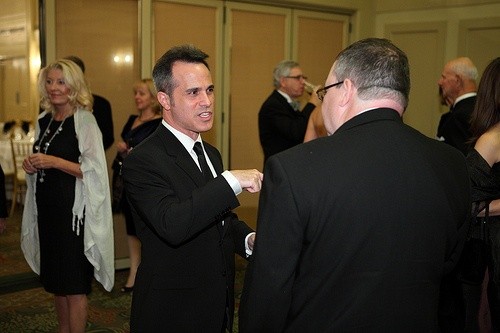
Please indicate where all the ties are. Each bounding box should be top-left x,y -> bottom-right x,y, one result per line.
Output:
193,143 -> 214,181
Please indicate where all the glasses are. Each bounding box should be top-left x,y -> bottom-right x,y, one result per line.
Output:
285,75 -> 308,81
316,81 -> 344,101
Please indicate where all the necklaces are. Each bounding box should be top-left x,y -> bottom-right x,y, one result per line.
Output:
138,113 -> 156,123
35,110 -> 73,182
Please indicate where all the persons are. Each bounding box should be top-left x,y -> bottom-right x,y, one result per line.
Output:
470,56 -> 500,333
111,79 -> 163,294
19,57 -> 115,333
60,55 -> 115,152
258,60 -> 324,160
121,47 -> 264,333
238,38 -> 472,333
436,56 -> 480,155
303,97 -> 327,143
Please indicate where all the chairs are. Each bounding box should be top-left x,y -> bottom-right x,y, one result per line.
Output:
9,140 -> 34,218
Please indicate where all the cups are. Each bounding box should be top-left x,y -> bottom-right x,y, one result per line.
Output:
304,83 -> 324,98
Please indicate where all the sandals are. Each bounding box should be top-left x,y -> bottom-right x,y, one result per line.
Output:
119,285 -> 134,294
